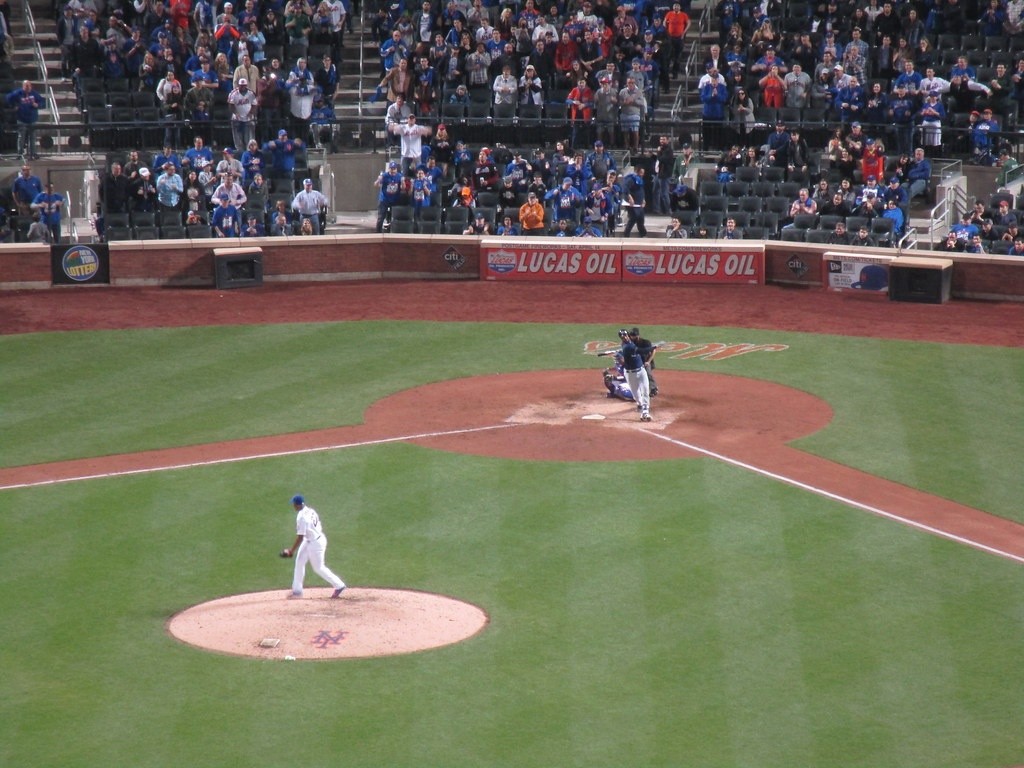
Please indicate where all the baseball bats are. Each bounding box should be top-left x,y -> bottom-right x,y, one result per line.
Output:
597,349 -> 619,357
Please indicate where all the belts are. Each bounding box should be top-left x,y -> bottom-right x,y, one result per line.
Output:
626,368 -> 642,373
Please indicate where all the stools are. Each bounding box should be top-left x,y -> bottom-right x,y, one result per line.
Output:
213,247 -> 264,290
889,256 -> 953,304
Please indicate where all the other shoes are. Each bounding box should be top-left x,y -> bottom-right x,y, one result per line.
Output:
637,405 -> 641,410
649,388 -> 658,397
641,412 -> 651,421
287,593 -> 302,599
331,585 -> 346,598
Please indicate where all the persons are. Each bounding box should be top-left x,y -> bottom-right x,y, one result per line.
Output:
5,80 -> 44,161
0,166 -> 64,243
603,328 -> 658,420
280,495 -> 346,599
0,0 -> 16,107
58,0 -> 354,238
366,0 -> 1024,256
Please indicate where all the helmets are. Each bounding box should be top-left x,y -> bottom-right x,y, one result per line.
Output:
619,330 -> 628,338
631,328 -> 639,335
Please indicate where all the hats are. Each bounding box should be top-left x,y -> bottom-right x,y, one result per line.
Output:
133,17 -> 1018,233
290,495 -> 304,505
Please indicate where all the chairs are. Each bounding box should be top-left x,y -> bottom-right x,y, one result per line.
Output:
0,0 -> 1024,257
